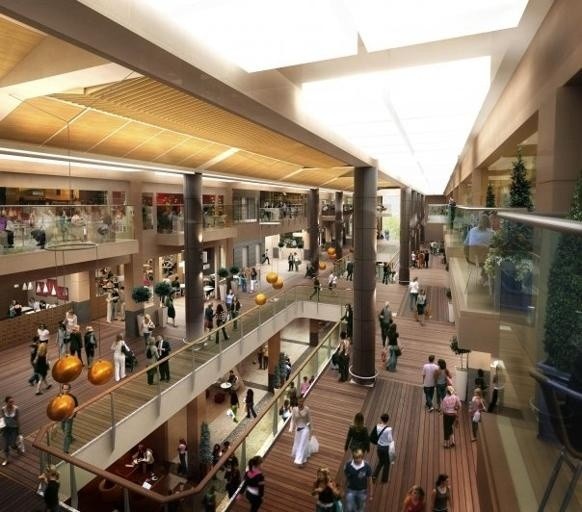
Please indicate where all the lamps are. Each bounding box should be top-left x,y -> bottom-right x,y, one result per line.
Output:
14,282 -> 56,296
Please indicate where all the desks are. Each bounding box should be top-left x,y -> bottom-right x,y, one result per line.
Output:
21,305 -> 33,313
220,382 -> 232,394
24,310 -> 35,314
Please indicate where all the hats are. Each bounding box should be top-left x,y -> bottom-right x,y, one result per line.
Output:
72,325 -> 79,331
86,326 -> 93,331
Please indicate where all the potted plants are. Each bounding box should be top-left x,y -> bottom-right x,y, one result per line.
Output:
449,334 -> 470,403
446,290 -> 456,323
272,353 -> 291,396
218,268 -> 229,302
155,281 -> 173,328
199,422 -> 214,478
230,265 -> 240,296
534,172 -> 582,445
480,144 -> 534,309
132,287 -> 152,337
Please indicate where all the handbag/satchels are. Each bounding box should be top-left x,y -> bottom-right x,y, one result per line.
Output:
395,347 -> 402,356
334,500 -> 344,512
371,426 -> 378,445
310,436 -> 319,453
90,338 -> 95,344
16,434 -> 25,453
0,417 -> 6,428
63,335 -> 70,343
149,325 -> 154,331
446,376 -> 453,386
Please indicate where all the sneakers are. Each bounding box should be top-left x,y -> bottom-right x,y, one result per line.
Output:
27,378 -> 52,395
2,460 -> 8,466
425,404 -> 440,412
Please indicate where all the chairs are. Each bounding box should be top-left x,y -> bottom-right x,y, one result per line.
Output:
526,367 -> 582,512
231,375 -> 239,391
97,478 -> 124,508
462,245 -> 493,298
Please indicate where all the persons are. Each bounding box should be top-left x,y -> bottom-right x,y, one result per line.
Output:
1,198 -> 506,512
